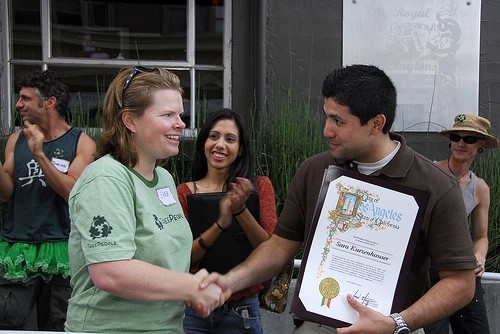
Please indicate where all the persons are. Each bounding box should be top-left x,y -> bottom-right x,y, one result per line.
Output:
0,67 -> 102,334
173,105 -> 281,334
61,63 -> 227,334
420,111 -> 495,334
184,63 -> 477,334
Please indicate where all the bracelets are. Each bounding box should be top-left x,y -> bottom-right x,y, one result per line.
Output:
214,220 -> 225,233
232,206 -> 246,217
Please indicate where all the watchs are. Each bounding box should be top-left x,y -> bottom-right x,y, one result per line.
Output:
388,311 -> 410,334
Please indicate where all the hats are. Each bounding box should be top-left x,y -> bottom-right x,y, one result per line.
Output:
439,113 -> 499,149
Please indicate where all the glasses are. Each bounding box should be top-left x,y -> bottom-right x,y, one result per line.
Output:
449,133 -> 482,144
123,66 -> 158,91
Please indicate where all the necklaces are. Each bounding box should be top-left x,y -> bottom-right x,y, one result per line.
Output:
448,157 -> 472,182
